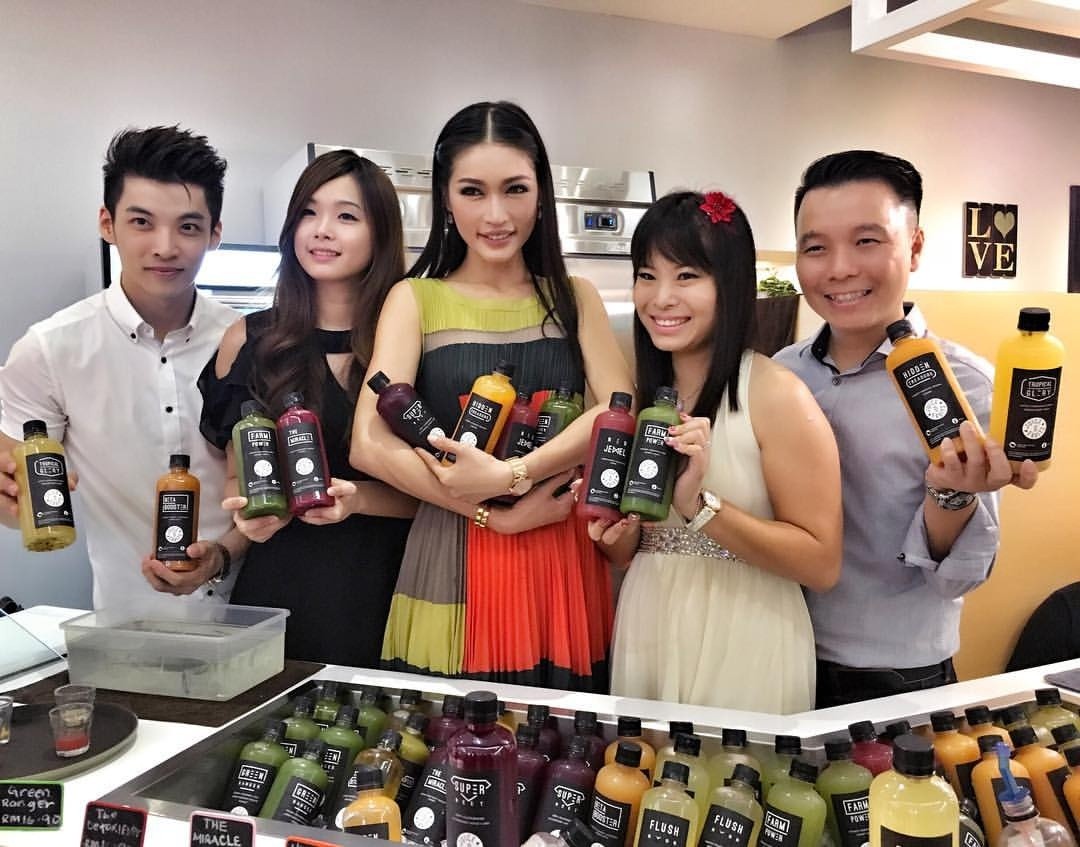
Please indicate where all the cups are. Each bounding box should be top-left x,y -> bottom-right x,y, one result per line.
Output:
0,696 -> 15,744
55,684 -> 95,728
50,703 -> 94,757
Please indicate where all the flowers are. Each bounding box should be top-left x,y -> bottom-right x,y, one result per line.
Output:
699,191 -> 736,223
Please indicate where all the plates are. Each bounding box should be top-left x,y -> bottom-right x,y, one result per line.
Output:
884,320 -> 986,467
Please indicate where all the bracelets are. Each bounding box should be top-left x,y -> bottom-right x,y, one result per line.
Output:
472,502 -> 489,529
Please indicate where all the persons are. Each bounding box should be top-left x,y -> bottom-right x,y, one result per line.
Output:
348,100 -> 637,693
196,149 -> 421,674
569,190 -> 842,715
0,123 -> 251,626
770,150 -> 1039,710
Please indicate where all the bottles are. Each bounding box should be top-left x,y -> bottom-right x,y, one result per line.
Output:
817,687 -> 1080,847
227,681 -> 557,847
575,392 -> 636,521
497,390 -> 539,504
990,307 -> 1062,473
275,392 -> 334,515
152,455 -> 201,571
366,370 -> 451,462
10,420 -> 77,551
521,711 -> 827,847
231,399 -> 290,519
532,378 -> 583,452
439,359 -> 517,466
619,386 -> 683,520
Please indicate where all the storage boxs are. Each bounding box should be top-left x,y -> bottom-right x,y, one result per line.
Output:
59,595 -> 293,698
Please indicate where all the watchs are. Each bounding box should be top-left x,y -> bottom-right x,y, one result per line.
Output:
683,488 -> 721,533
208,543 -> 232,589
927,486 -> 976,510
507,454 -> 532,497
